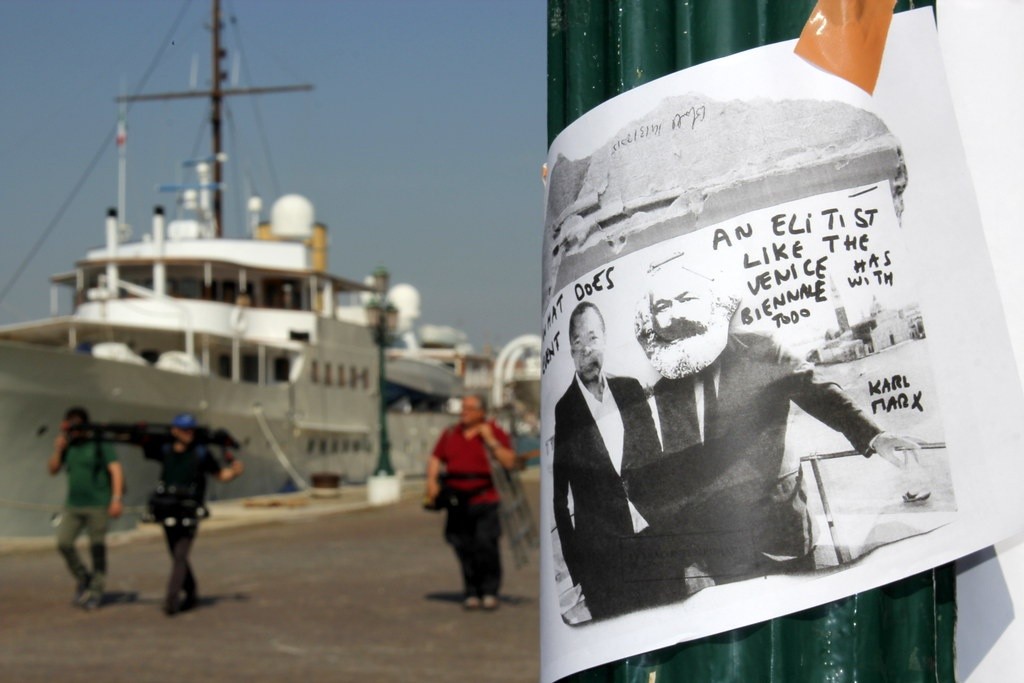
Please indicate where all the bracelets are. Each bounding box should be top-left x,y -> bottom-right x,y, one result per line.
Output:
488,443 -> 500,449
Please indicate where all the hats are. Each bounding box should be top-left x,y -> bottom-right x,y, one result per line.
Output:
173,414 -> 197,428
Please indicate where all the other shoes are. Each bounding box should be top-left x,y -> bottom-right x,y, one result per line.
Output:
463,595 -> 497,609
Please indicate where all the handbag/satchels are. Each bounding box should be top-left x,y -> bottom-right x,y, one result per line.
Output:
439,487 -> 468,507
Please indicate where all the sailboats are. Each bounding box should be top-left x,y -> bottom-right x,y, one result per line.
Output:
0,1 -> 541,542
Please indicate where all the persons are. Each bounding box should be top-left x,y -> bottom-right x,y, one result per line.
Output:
423,390 -> 519,611
49,405 -> 123,609
140,410 -> 244,617
553,246 -> 927,622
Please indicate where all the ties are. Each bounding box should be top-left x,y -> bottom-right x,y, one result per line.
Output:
700,364 -> 719,447
